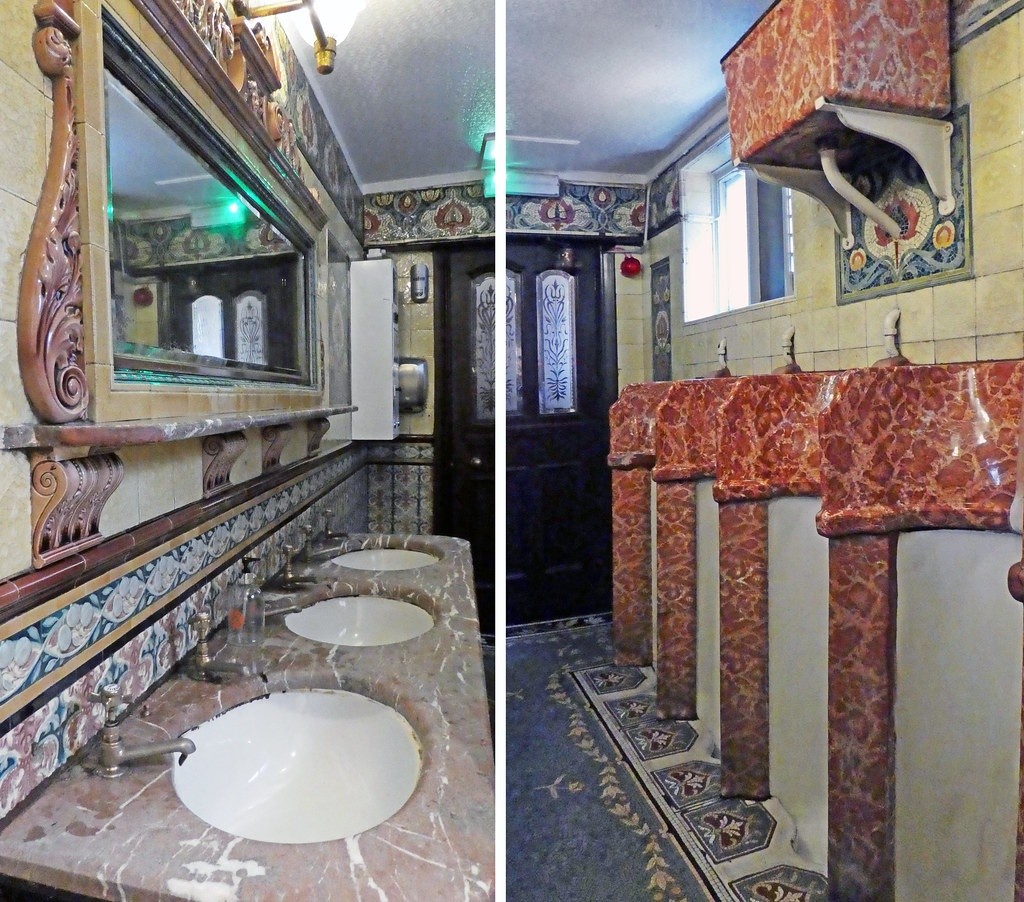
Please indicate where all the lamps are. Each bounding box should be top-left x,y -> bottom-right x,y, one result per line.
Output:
232,0 -> 366,75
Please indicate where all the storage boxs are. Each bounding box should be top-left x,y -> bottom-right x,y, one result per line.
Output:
719,0 -> 951,163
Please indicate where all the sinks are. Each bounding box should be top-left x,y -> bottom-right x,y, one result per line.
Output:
330,548 -> 440,571
284,593 -> 435,646
171,686 -> 426,845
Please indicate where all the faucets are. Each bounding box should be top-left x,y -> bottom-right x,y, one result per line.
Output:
276,544 -> 318,588
320,509 -> 350,541
88,683 -> 197,779
187,611 -> 252,682
298,523 -> 346,562
234,572 -> 302,630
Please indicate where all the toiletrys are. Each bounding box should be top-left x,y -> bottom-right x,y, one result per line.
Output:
225,557 -> 266,646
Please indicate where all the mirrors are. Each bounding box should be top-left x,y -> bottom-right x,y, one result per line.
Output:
77,0 -> 328,421
